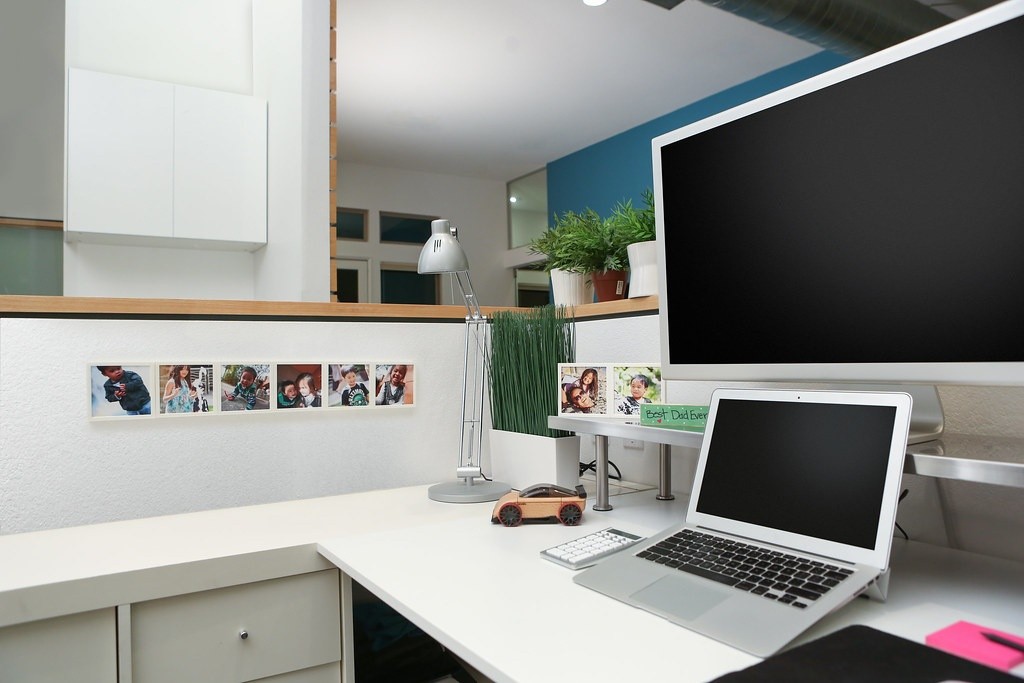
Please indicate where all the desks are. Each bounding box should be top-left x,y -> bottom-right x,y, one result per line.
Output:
0,482 -> 1024,683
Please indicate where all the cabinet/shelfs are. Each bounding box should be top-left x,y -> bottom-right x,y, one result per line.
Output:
63,68 -> 267,251
0,568 -> 354,683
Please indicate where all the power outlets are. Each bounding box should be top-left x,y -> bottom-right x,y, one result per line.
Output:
622,439 -> 643,450
616,281 -> 623,295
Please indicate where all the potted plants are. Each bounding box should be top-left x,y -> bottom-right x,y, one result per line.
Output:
533,212 -> 601,306
602,191 -> 656,300
479,304 -> 580,493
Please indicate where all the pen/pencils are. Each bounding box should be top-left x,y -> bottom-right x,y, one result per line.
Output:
981,631 -> 1024,654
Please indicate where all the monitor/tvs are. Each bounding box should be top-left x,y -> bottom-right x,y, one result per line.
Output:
652,0 -> 1024,445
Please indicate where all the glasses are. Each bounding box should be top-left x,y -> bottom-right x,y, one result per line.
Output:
575,390 -> 585,401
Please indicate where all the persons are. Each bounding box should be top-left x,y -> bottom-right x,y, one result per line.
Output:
97,366 -> 151,415
617,374 -> 652,414
376,365 -> 407,405
162,365 -> 197,413
340,365 -> 369,406
224,366 -> 257,410
294,372 -> 319,408
561,369 -> 606,414
277,380 -> 305,408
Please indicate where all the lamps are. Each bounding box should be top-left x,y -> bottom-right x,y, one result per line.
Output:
420,222 -> 511,503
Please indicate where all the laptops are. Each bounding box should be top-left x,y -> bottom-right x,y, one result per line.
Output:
572,389 -> 914,660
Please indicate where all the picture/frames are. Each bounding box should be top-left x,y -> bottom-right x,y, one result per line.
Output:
156,363 -> 216,418
372,361 -> 418,409
610,364 -> 666,414
326,362 -> 372,411
558,362 -> 609,414
275,363 -> 326,412
217,362 -> 274,415
85,363 -> 156,421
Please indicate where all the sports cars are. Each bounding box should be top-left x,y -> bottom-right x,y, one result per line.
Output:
490,483 -> 588,528
